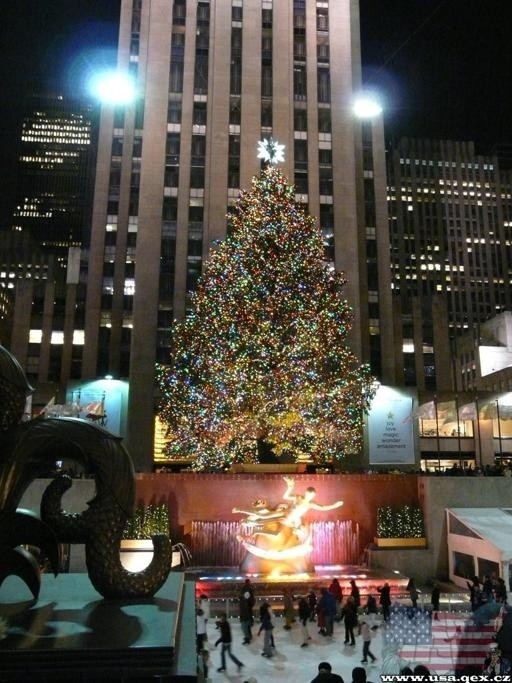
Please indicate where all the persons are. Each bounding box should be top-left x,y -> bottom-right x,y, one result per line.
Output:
232,475 -> 344,528
375,581 -> 392,624
395,667 -> 413,682
450,573 -> 511,682
387,604 -> 496,671
411,664 -> 434,682
404,576 -> 419,608
406,459 -> 512,477
197,574 -> 378,683
430,583 -> 441,620
